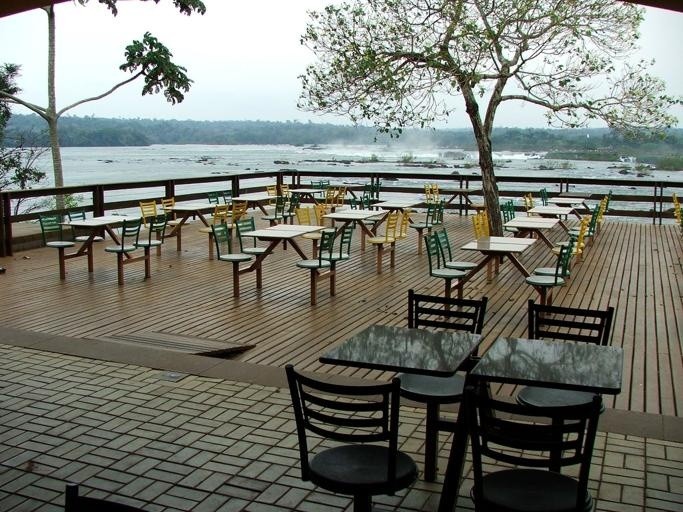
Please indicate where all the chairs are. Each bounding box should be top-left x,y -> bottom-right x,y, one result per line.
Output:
282,287 -> 624,511
37,208 -> 171,286
500,188 -> 612,269
61,479 -> 149,512
672,192 -> 682,233
211,216 -> 354,306
139,196 -> 247,261
423,227 -> 575,326
208,178 -> 445,275
